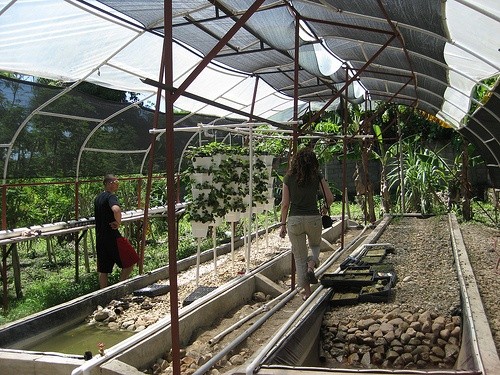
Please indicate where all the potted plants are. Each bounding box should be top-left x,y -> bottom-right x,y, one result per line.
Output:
174,142 -> 274,237
316,121 -> 340,146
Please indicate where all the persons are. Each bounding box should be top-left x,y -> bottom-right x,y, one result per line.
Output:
93,174 -> 136,289
279,147 -> 334,301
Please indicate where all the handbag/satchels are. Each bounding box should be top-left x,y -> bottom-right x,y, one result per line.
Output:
322,214 -> 334,229
113,230 -> 141,268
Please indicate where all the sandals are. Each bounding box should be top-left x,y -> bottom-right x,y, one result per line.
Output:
306,267 -> 318,284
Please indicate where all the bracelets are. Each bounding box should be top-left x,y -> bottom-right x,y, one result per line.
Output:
324,205 -> 329,210
282,223 -> 287,226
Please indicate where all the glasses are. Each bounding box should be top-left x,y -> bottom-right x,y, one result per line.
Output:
107,180 -> 119,183
